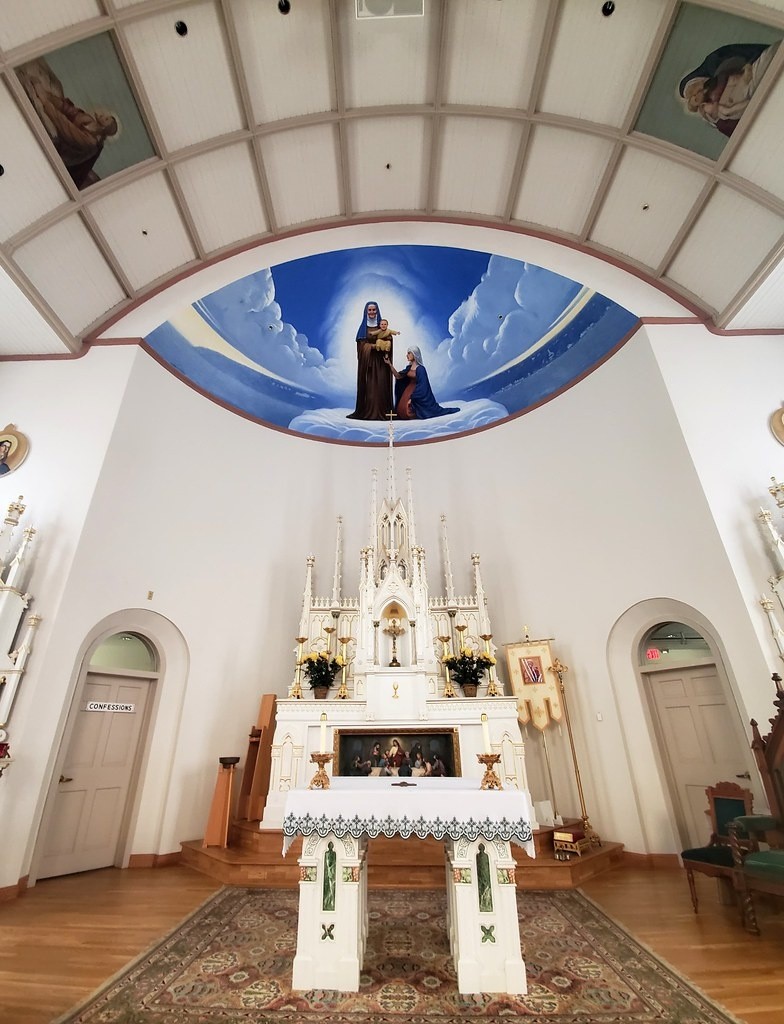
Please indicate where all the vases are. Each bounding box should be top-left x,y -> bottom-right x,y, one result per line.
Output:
461,684 -> 477,697
314,686 -> 328,699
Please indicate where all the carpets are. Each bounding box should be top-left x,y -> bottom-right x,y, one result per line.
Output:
48,883 -> 748,1024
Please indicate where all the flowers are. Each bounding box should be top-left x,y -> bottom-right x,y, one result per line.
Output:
294,652 -> 344,691
441,644 -> 498,688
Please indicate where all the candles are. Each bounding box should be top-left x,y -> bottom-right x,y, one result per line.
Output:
343,643 -> 346,662
445,664 -> 450,682
443,642 -> 448,656
482,722 -> 492,756
320,722 -> 325,755
297,665 -> 300,683
485,641 -> 490,655
460,631 -> 464,648
489,667 -> 493,681
342,667 -> 345,683
327,633 -> 330,651
298,642 -> 303,661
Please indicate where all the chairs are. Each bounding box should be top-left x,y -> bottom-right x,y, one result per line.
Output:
680,782 -> 760,929
727,672 -> 784,936
